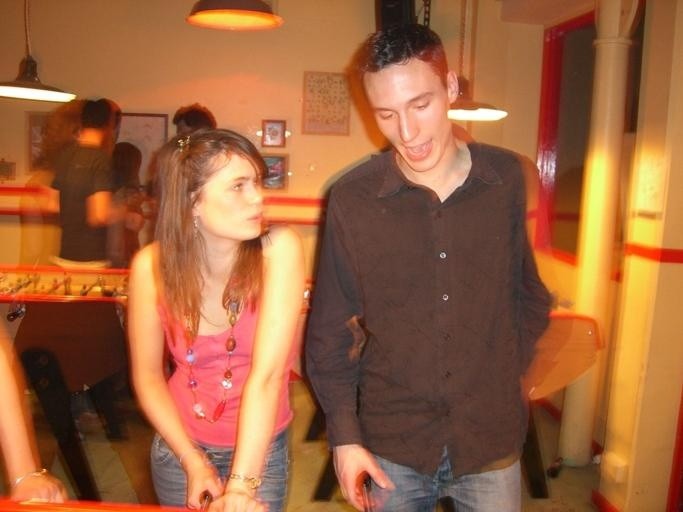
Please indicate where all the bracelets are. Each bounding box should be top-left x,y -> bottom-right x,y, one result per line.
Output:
10,468 -> 49,490
224,471 -> 264,492
181,450 -> 202,468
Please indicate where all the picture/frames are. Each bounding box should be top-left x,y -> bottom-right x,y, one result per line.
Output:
28,110 -> 172,174
300,69 -> 351,136
256,116 -> 291,194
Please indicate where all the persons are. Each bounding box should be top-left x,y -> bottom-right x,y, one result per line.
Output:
0,325 -> 71,505
105,141 -> 147,264
126,128 -> 309,512
305,23 -> 551,512
35,97 -> 145,272
168,103 -> 217,135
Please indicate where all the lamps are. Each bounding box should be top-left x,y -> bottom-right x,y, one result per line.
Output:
184,1 -> 285,31
440,0 -> 509,124
1,0 -> 77,106
